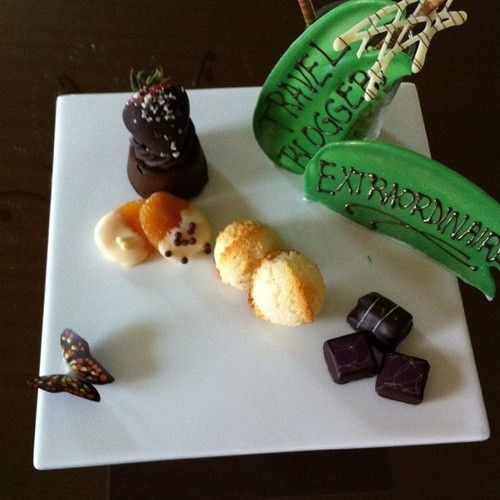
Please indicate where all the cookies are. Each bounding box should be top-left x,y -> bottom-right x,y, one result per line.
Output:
301,138 -> 500,302
251,1 -> 413,172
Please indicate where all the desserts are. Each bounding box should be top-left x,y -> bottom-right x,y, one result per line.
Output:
121,61 -> 208,202
324,291 -> 430,405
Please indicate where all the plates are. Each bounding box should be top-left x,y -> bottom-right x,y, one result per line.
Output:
32,82 -> 491,470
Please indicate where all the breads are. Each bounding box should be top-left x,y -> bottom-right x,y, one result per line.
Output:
215,220 -> 285,290
249,249 -> 325,329
